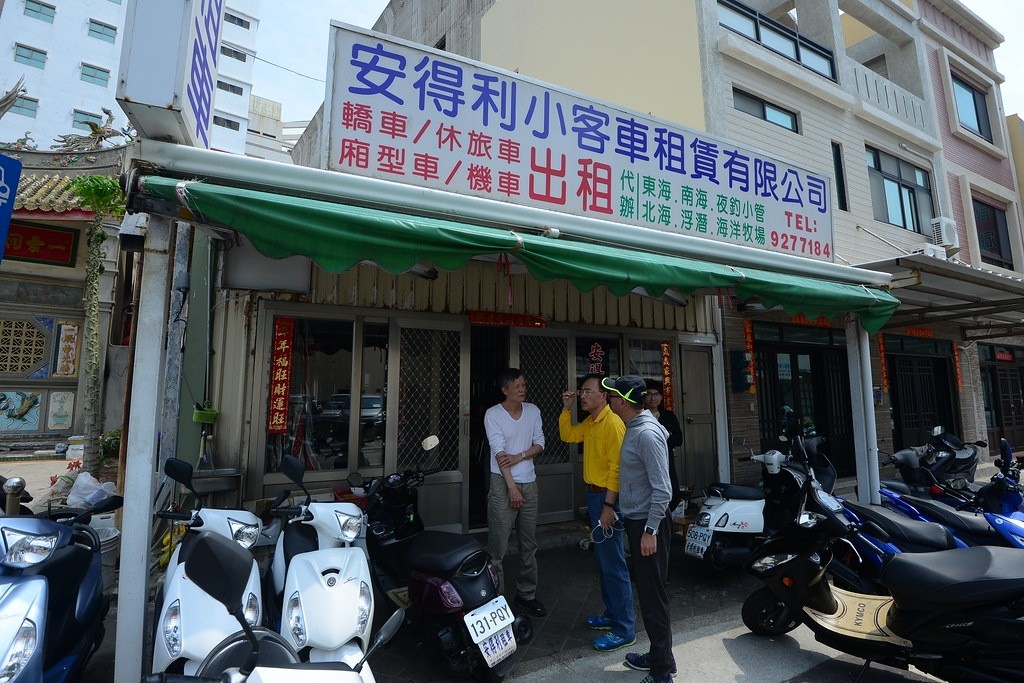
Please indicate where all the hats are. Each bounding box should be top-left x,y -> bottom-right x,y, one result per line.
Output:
602,375 -> 648,404
646,379 -> 661,392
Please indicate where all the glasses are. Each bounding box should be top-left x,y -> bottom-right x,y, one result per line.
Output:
645,390 -> 660,398
577,389 -> 606,397
608,393 -> 624,399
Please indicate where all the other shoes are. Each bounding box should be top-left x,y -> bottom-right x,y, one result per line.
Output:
514,594 -> 546,615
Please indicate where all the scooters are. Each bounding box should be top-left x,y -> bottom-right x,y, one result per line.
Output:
262,455 -> 402,683
346,434 -> 533,683
827,425 -> 1024,597
146,531 -> 405,683
684,405 -> 837,569
0,496 -> 123,682
323,437 -> 375,469
741,435 -> 1024,683
146,458 -> 291,677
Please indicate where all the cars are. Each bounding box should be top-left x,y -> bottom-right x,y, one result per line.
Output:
290,401 -> 323,427
329,394 -> 350,403
342,395 -> 384,431
319,401 -> 344,421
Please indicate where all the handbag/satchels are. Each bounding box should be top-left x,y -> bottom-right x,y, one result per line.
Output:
67,471 -> 102,508
87,481 -> 116,508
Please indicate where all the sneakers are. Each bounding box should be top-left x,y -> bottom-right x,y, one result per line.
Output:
585,613 -> 613,630
626,648 -> 677,677
640,672 -> 674,683
593,632 -> 636,652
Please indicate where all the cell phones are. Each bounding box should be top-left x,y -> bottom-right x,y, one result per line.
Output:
598,511 -> 619,527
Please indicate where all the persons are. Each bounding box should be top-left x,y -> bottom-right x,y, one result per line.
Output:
644,380 -> 683,585
558,373 -> 637,652
601,376 -> 677,683
484,368 -> 548,618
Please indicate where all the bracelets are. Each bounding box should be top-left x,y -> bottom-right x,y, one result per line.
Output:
604,501 -> 615,507
520,453 -> 527,459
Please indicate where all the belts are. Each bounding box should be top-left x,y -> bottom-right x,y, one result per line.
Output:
583,484 -> 607,494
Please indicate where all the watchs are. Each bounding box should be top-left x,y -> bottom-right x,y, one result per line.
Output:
644,525 -> 658,535
